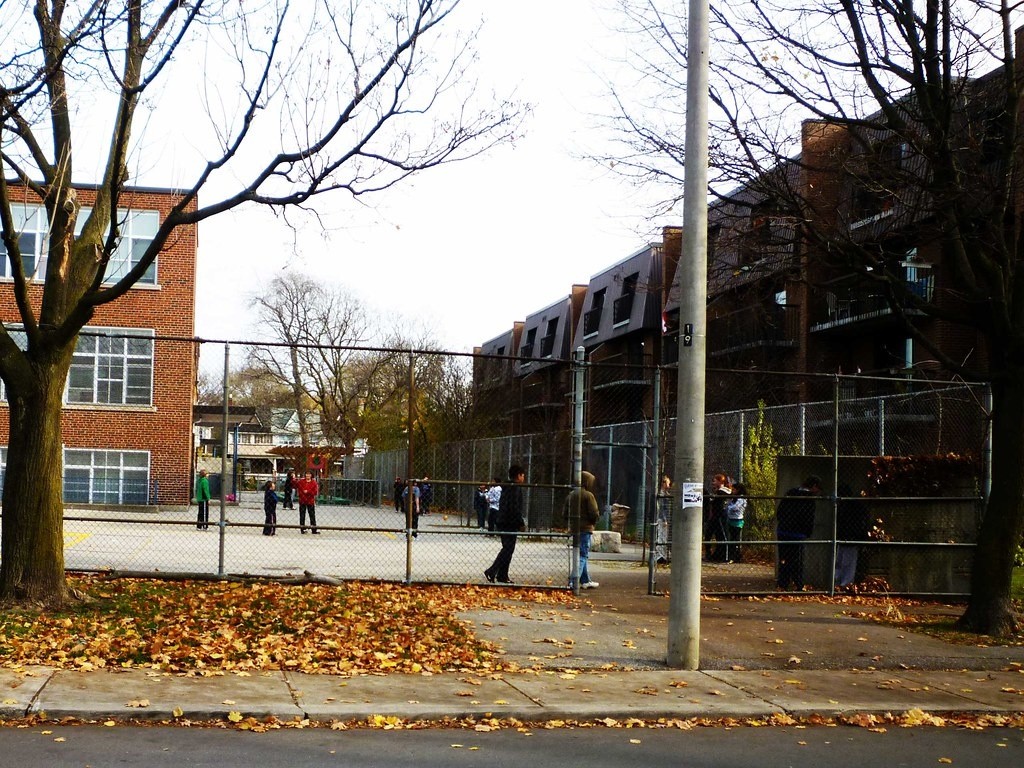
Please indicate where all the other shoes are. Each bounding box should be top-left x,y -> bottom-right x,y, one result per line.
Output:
196,528 -> 204,531
702,553 -> 740,564
205,528 -> 211,531
312,531 -> 320,534
301,530 -> 307,534
291,507 -> 297,510
282,507 -> 288,510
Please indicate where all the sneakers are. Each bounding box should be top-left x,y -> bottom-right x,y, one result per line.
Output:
583,581 -> 599,589
484,570 -> 495,583
497,577 -> 514,584
568,580 -> 582,590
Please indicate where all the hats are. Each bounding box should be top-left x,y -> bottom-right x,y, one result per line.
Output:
806,475 -> 824,492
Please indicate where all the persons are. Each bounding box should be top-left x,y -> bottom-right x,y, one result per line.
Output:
393,475 -> 432,516
291,471 -> 321,534
473,483 -> 489,535
485,477 -> 502,537
562,471 -> 600,590
484,465 -> 526,584
196,469 -> 211,531
656,474 -> 673,567
402,480 -> 421,541
775,474 -> 822,592
283,470 -> 296,510
263,480 -> 278,537
703,473 -> 747,566
